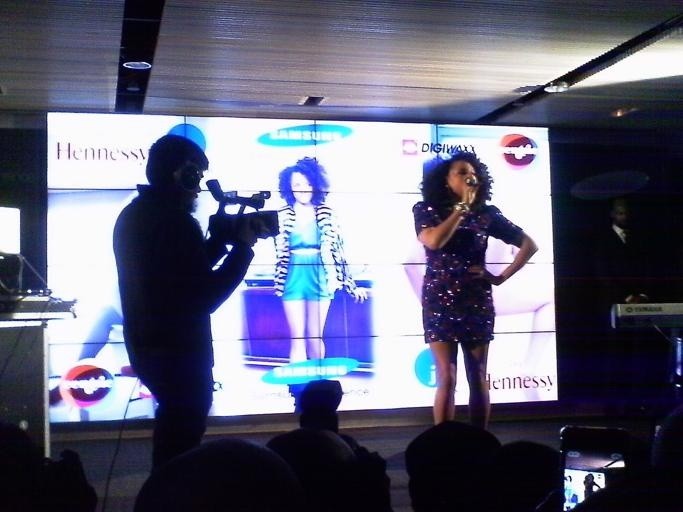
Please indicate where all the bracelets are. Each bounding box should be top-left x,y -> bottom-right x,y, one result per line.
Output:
453,200 -> 471,216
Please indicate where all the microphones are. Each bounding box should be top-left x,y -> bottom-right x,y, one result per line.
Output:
466,175 -> 481,190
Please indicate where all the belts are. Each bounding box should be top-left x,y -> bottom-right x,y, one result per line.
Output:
290,248 -> 321,255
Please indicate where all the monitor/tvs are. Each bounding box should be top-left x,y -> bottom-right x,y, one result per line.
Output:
563,465 -> 608,512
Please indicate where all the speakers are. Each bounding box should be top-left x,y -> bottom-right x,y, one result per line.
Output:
557,424 -> 632,471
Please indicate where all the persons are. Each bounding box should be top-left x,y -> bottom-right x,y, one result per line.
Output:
0,405 -> 682,510
583,197 -> 655,306
115,134 -> 262,476
412,151 -> 539,430
268,153 -> 368,407
